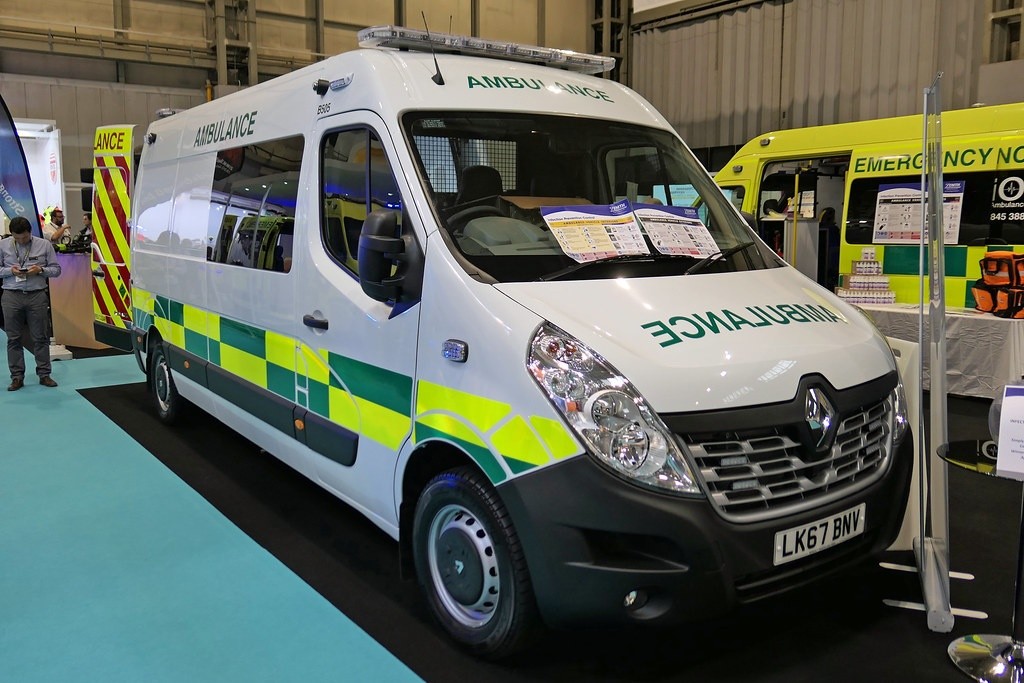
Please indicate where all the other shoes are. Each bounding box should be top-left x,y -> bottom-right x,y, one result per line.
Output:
8,380 -> 23,391
40,376 -> 57,387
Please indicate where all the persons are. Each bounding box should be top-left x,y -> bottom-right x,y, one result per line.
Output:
0,217 -> 61,391
79,213 -> 93,242
42,210 -> 72,245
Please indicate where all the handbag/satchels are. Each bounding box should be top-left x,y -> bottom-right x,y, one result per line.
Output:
971,279 -> 1024,319
979,251 -> 1024,290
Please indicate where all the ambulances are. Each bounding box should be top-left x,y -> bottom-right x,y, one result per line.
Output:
689,99 -> 1024,447
90,23 -> 914,667
211,182 -> 401,280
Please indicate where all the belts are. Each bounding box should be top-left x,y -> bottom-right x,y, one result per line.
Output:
4,289 -> 42,294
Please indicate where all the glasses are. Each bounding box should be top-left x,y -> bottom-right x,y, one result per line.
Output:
54,216 -> 65,218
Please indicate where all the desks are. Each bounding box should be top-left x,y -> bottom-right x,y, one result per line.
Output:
936,439 -> 1024,683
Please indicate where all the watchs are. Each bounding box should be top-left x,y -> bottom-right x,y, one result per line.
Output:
39,267 -> 44,275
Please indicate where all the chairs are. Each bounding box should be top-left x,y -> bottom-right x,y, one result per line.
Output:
454,165 -> 505,210
817,206 -> 839,253
531,166 -> 573,199
